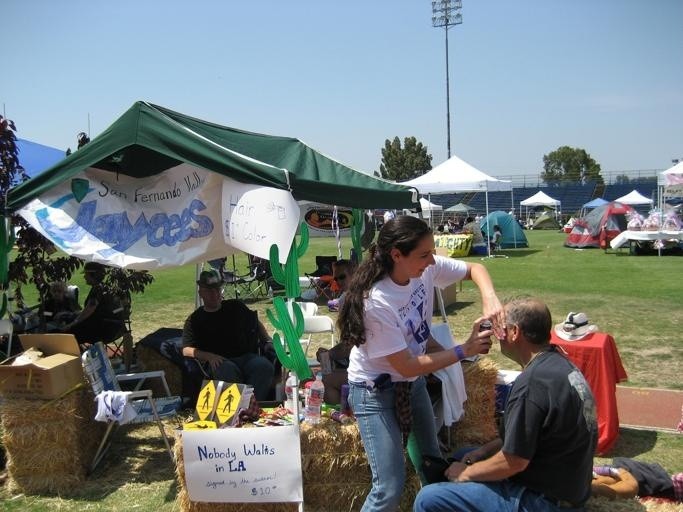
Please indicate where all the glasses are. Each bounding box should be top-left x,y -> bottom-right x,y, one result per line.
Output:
334,275 -> 346,281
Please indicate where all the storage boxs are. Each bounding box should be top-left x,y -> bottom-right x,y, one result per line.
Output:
0,332 -> 84,401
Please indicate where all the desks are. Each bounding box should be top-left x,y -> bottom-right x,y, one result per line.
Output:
549,328 -> 612,451
628,230 -> 683,257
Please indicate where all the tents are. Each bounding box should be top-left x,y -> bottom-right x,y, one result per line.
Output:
474,210 -> 529,248
530,211 -> 561,230
562,199 -> 640,249
562,217 -> 578,233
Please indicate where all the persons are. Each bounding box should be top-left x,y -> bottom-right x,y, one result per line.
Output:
433,216 -> 503,254
382,209 -> 395,226
528,215 -> 533,228
518,217 -> 523,229
179,270 -> 274,405
65,261 -> 120,343
409,294 -> 599,511
315,340 -> 351,407
37,280 -> 82,324
337,214 -> 508,511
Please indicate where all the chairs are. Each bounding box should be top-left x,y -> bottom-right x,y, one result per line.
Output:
14,285 -> 82,333
485,233 -> 504,258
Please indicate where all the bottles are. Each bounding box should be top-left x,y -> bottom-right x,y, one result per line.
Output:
304,371 -> 324,425
283,371 -> 300,409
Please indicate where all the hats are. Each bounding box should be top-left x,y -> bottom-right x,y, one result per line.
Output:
555,312 -> 598,342
80,262 -> 104,273
196,270 -> 227,287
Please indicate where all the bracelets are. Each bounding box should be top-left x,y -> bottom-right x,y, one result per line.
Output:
453,344 -> 465,360
